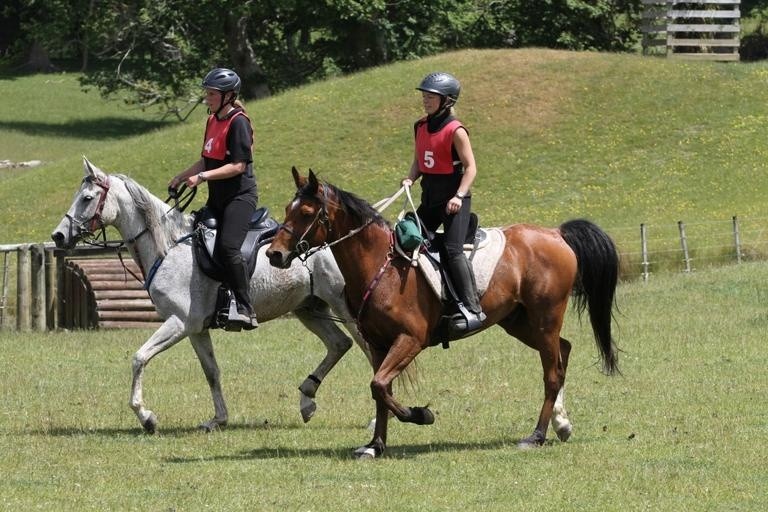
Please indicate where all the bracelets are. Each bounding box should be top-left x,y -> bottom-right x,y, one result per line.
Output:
456,192 -> 464,200
175,176 -> 182,185
197,172 -> 206,184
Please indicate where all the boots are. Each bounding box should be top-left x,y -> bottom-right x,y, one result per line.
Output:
218,256 -> 259,329
451,254 -> 485,330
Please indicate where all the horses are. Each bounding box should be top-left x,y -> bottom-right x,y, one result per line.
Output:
48,154 -> 425,434
265,164 -> 624,462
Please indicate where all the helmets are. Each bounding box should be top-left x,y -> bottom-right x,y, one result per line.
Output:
201,67 -> 241,94
416,72 -> 460,101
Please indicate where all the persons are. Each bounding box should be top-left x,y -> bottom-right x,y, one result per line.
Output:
399,72 -> 488,329
166,67 -> 259,332
